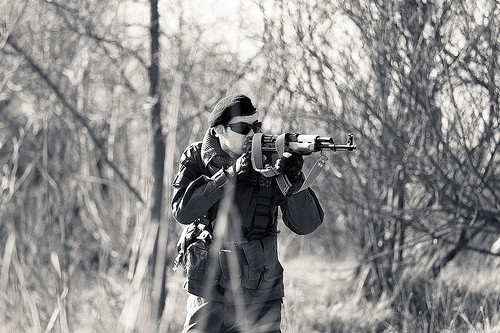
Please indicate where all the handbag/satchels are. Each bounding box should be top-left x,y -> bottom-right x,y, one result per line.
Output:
176,223 -> 216,298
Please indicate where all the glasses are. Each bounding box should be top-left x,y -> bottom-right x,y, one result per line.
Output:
225,120 -> 262,136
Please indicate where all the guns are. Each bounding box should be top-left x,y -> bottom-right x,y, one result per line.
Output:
244,132 -> 357,198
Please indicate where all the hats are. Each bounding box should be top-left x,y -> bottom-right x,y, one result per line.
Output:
208,94 -> 258,128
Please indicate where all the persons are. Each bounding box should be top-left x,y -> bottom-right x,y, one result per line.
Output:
169,94 -> 325,333
